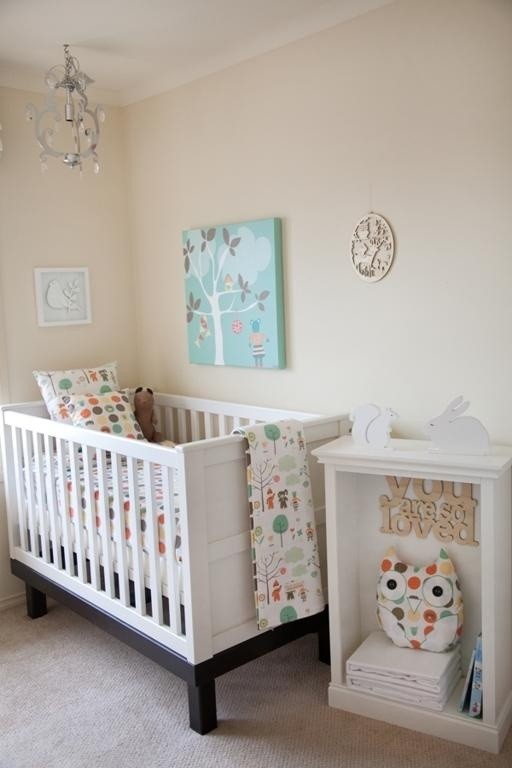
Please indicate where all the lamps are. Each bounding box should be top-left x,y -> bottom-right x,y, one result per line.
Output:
25,43 -> 108,176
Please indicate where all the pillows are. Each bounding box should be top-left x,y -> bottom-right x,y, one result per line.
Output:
32,360 -> 121,452
60,388 -> 150,468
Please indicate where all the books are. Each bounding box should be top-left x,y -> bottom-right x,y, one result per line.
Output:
456,648 -> 476,714
466,631 -> 482,718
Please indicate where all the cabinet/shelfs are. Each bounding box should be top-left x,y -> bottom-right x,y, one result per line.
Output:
310,393 -> 512,754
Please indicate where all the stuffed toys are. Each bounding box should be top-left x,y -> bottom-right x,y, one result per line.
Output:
128,385 -> 165,444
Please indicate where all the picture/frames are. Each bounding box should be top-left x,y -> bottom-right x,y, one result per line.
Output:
33,266 -> 92,328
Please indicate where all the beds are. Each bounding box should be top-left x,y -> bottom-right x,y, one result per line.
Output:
0,388 -> 353,737
